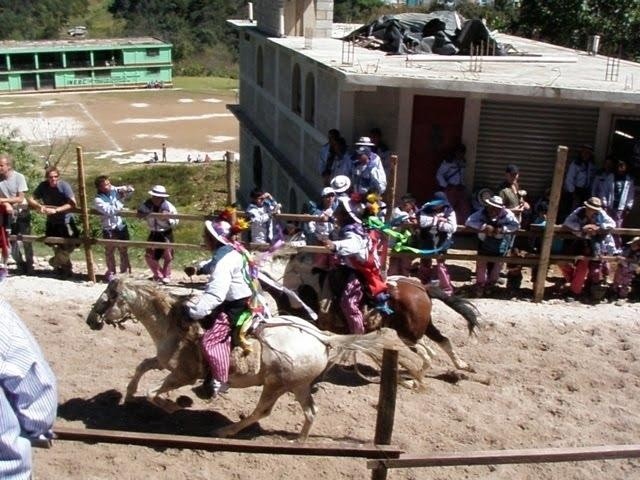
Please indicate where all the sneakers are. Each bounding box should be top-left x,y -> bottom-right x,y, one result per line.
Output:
163,275 -> 172,284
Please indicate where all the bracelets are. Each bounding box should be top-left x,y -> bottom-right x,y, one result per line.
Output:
54,208 -> 58,213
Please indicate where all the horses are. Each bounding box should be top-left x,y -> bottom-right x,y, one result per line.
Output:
85,270 -> 431,445
250,230 -> 485,374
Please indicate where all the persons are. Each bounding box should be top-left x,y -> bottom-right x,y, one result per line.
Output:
246,125 -> 639,306
0,292 -> 58,480
94,176 -> 137,278
27,166 -> 76,280
150,151 -> 158,162
162,142 -> 167,163
137,182 -> 179,284
187,153 -> 212,163
179,205 -> 257,399
0,155 -> 33,275
322,197 -> 379,335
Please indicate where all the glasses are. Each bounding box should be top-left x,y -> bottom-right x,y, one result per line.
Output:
46,175 -> 59,179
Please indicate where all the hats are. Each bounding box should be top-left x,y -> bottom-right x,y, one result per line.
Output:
584,196 -> 604,213
338,195 -> 368,225
355,136 -> 375,147
575,143 -> 595,154
147,184 -> 170,200
354,146 -> 372,158
505,164 -> 520,174
329,174 -> 352,193
202,219 -> 239,252
484,194 -> 506,209
320,186 -> 337,198
428,191 -> 451,207
